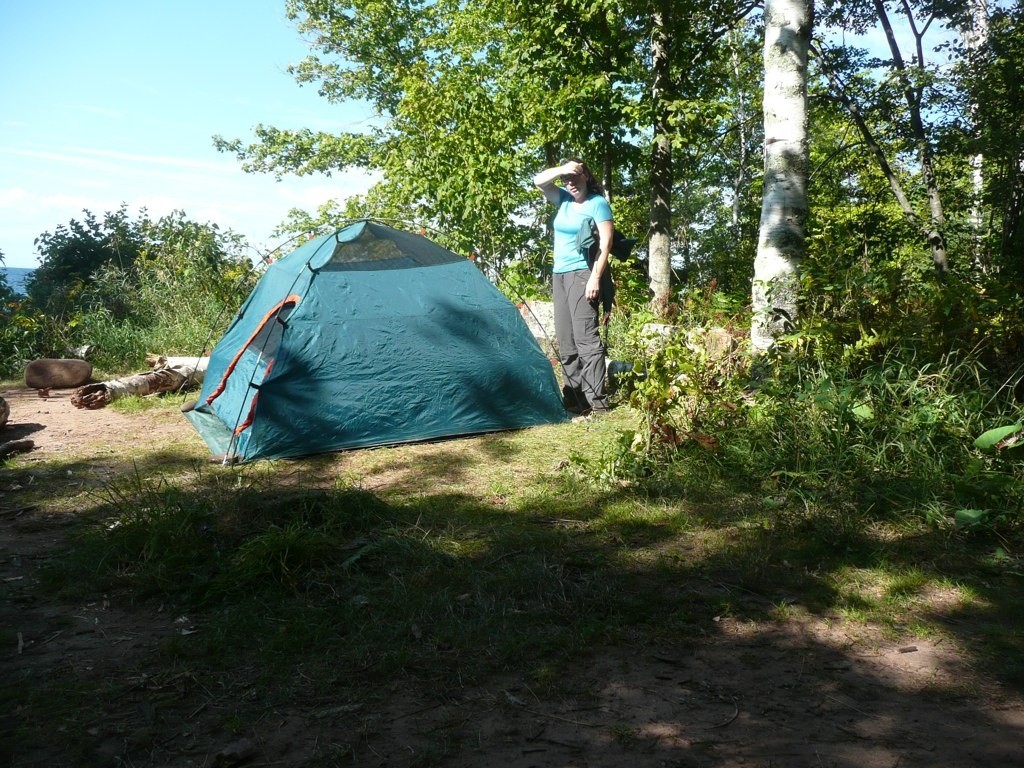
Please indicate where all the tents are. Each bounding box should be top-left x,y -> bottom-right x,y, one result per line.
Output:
181,217 -> 585,472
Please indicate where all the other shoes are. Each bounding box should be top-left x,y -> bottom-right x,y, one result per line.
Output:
561,396 -> 578,409
588,407 -> 610,418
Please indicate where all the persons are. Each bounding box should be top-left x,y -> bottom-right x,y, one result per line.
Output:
534,157 -> 615,419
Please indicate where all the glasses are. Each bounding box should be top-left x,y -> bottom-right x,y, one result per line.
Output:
563,173 -> 581,183
